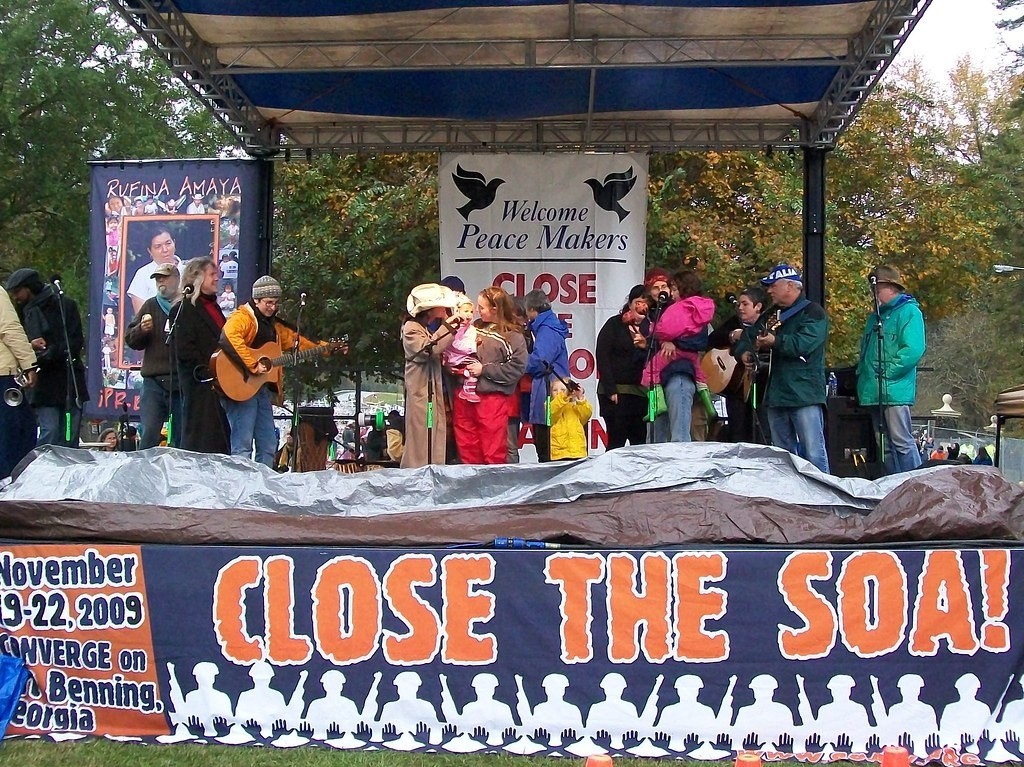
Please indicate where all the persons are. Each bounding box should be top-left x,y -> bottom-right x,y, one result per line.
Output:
98,426 -> 140,451
218,276 -> 348,469
596,265 -> 830,475
856,265 -> 926,475
0,268 -> 91,481
273,410 -> 405,472
400,276 -> 592,469
911,429 -> 993,466
102,193 -> 239,388
169,255 -> 231,456
125,263 -> 203,450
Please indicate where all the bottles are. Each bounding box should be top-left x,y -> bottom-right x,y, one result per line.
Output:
828,372 -> 837,395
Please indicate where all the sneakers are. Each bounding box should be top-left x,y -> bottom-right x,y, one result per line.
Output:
459,389 -> 481,403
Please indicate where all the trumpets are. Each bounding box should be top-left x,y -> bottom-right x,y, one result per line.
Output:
4,366 -> 41,407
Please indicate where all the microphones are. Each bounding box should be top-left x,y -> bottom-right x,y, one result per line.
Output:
454,317 -> 463,323
868,273 -> 879,285
301,293 -> 307,306
50,274 -> 65,296
725,293 -> 738,304
184,283 -> 195,294
658,291 -> 668,302
569,379 -> 585,395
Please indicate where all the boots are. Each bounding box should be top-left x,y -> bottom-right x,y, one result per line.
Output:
698,383 -> 718,426
643,383 -> 667,422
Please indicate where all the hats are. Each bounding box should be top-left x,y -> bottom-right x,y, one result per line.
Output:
875,265 -> 906,290
759,265 -> 801,285
453,290 -> 473,307
150,263 -> 180,279
644,266 -> 669,289
5,268 -> 39,289
407,283 -> 458,317
252,275 -> 282,299
191,194 -> 204,199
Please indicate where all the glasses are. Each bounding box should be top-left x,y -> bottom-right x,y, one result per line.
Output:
262,298 -> 280,308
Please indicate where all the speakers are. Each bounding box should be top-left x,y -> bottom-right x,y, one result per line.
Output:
825,396 -> 881,481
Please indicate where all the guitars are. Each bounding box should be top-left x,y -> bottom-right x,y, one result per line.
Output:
209,334 -> 350,402
698,348 -> 738,398
742,309 -> 783,404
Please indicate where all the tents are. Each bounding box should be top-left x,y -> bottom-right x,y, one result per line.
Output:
994,384 -> 1024,467
